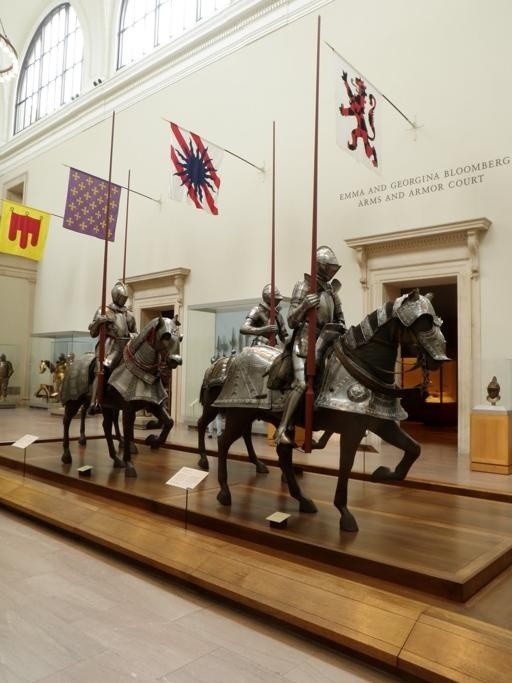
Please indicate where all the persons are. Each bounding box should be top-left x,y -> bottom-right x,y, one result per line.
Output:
274,246 -> 347,447
206,414 -> 223,438
240,282 -> 287,349
89,282 -> 138,413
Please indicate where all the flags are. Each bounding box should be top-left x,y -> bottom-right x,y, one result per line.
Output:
161,118 -> 226,218
0,199 -> 52,263
62,166 -> 123,243
327,41 -> 385,175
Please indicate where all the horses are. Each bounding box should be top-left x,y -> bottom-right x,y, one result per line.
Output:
197,287 -> 453,533
60,314 -> 184,478
